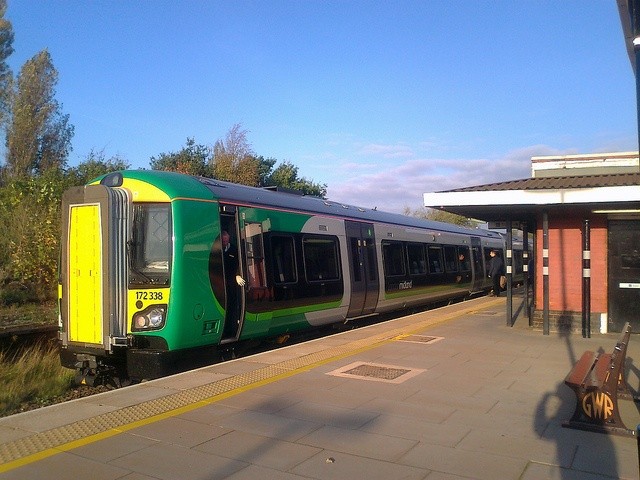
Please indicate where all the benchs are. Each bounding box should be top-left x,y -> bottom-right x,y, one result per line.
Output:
560,322 -> 640,438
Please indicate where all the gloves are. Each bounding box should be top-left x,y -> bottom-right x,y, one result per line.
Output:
236,276 -> 246,286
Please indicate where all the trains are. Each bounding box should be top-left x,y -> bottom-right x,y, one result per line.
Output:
57,170 -> 533,386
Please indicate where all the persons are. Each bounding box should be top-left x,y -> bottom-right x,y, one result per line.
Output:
456,254 -> 466,283
222,231 -> 245,338
488,249 -> 503,297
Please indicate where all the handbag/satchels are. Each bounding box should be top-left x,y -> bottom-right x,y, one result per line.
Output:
500,276 -> 507,289
